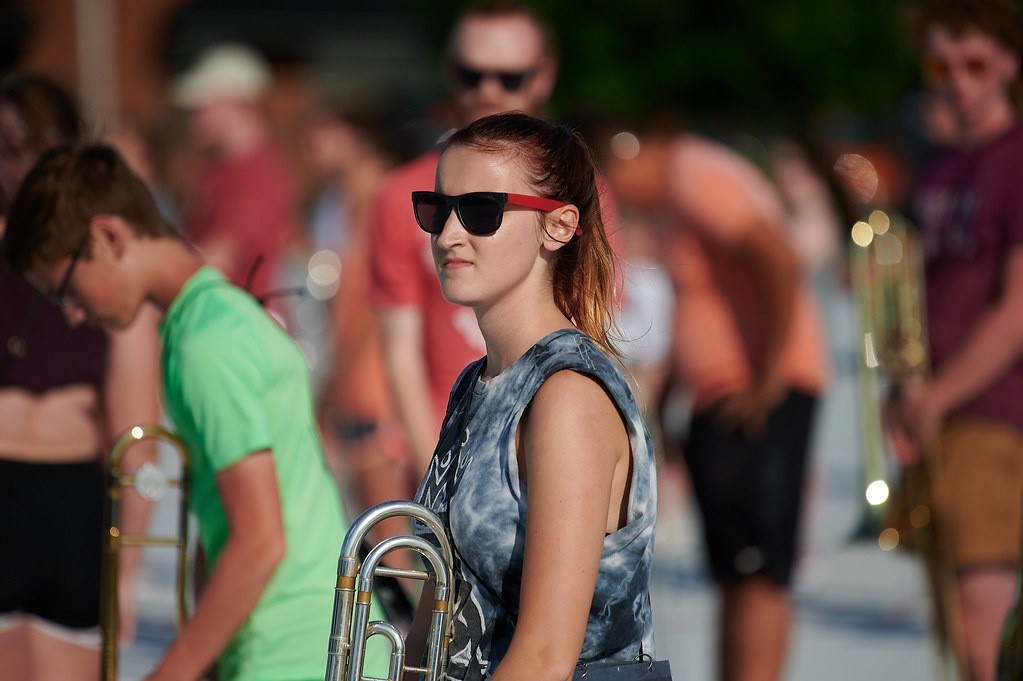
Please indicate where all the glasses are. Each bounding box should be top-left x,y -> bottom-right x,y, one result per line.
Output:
411,191 -> 583,236
48,232 -> 89,308
452,63 -> 532,92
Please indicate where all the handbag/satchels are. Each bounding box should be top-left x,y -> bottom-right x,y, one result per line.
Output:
572,654 -> 672,681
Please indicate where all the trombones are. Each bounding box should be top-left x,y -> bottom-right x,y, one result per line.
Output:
854,210 -> 973,681
101,424 -> 191,681
325,498 -> 455,681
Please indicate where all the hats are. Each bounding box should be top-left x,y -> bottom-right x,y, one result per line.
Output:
169,42 -> 268,106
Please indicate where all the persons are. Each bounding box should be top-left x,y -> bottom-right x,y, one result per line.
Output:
0,11 -> 841,680
879,1 -> 1023,681
0,135 -> 395,680
394,108 -> 658,680
